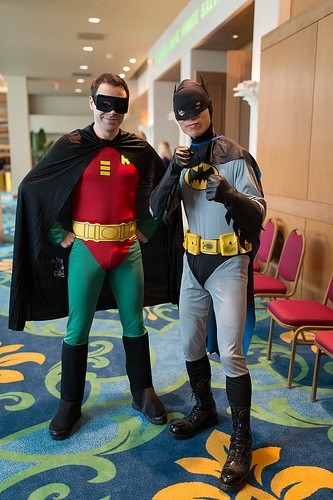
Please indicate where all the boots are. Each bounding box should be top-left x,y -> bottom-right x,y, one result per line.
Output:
49,339 -> 88,440
219,400 -> 252,491
123,329 -> 167,424
167,377 -> 218,437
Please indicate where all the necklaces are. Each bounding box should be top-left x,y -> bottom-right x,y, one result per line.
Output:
189,134 -> 226,148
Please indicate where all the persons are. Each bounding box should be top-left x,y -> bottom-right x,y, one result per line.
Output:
8,71 -> 185,441
148,75 -> 268,494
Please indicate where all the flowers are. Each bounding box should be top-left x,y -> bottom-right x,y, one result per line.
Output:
232,80 -> 260,113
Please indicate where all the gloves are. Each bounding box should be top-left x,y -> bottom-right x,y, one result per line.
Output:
150,146 -> 195,221
205,174 -> 262,243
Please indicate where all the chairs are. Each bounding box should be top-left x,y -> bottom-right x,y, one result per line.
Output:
253,217 -> 333,402
